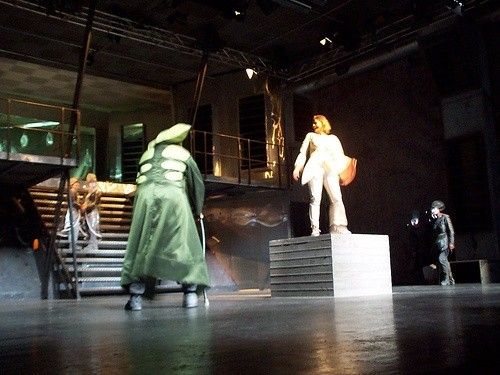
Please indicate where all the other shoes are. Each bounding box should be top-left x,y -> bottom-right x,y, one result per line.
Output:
311,226 -> 321,236
125,296 -> 142,311
329,225 -> 351,234
182,293 -> 197,307
441,279 -> 450,285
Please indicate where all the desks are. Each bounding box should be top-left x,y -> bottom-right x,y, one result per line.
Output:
439,259 -> 490,284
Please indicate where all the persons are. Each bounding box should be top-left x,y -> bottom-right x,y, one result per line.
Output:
292,114 -> 357,236
62,177 -> 82,254
82,173 -> 101,251
118,122 -> 206,311
429,200 -> 455,285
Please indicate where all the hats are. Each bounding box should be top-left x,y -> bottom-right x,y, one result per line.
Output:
148,123 -> 191,149
70,177 -> 79,186
86,174 -> 96,182
